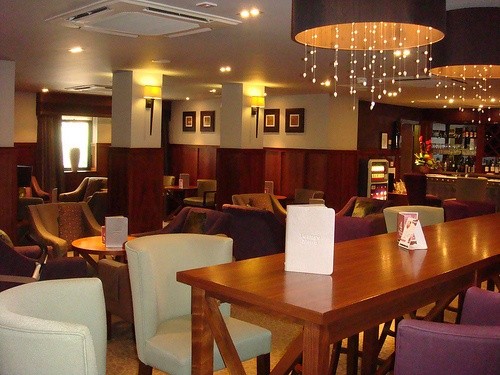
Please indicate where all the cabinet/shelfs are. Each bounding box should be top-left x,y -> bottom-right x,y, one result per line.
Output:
430,123 -> 482,173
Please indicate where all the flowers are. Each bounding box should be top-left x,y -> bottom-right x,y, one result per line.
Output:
414,135 -> 441,170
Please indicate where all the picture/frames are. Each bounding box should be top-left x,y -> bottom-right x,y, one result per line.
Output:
285,108 -> 305,133
264,108 -> 280,133
182,111 -> 197,133
199,110 -> 216,132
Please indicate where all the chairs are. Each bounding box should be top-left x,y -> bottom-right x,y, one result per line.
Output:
0,174 -> 500,375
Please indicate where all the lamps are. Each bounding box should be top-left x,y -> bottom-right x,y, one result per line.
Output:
252,96 -> 265,138
290,0 -> 500,125
144,84 -> 162,135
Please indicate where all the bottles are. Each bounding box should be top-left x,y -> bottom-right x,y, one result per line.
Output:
375,187 -> 379,196
470,127 -> 473,138
379,186 -> 383,195
490,159 -> 500,174
465,127 -> 469,137
474,127 -> 477,138
484,160 -> 489,173
384,186 -> 387,195
444,155 -> 476,174
436,154 -> 440,165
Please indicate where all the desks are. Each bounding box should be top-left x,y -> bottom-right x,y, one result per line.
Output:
164,185 -> 198,223
177,211 -> 500,375
388,191 -> 408,196
70,234 -> 137,279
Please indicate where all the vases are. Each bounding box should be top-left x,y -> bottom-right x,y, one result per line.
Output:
69,147 -> 80,172
418,165 -> 431,174
